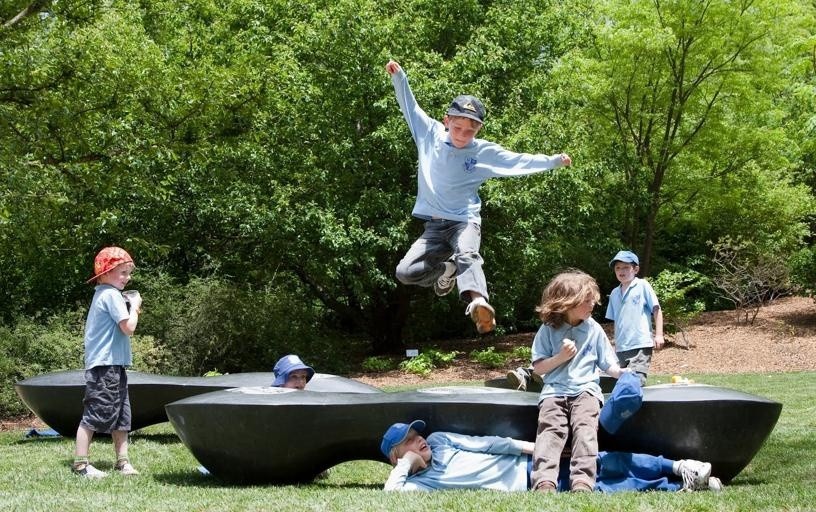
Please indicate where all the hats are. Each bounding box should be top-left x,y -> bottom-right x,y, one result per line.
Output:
84,246 -> 133,283
380,419 -> 426,456
609,250 -> 640,268
447,94 -> 487,125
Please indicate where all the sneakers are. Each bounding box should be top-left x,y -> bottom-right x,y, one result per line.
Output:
114,462 -> 139,475
73,464 -> 108,477
537,482 -> 556,495
708,476 -> 723,493
678,459 -> 712,494
571,480 -> 591,494
464,299 -> 497,334
434,255 -> 458,296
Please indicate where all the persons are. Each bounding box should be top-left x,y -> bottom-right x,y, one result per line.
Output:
531,272 -> 632,493
380,420 -> 724,493
385,60 -> 572,333
70,248 -> 142,480
508,365 -> 543,391
605,251 -> 664,387
